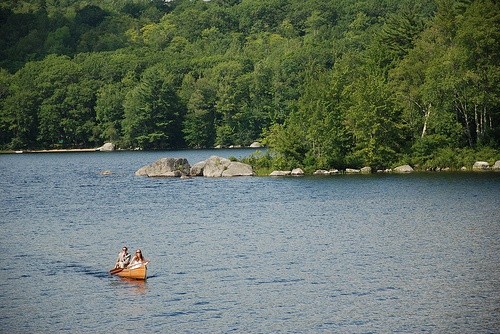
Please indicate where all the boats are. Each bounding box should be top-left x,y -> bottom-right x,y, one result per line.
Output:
109,261 -> 149,279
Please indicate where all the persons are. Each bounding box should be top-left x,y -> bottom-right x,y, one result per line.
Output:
128,249 -> 146,268
115,246 -> 131,270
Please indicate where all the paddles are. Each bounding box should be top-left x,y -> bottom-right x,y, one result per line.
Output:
110,259 -> 149,275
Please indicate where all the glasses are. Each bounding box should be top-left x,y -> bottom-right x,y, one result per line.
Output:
137,252 -> 141,254
123,250 -> 127,251
